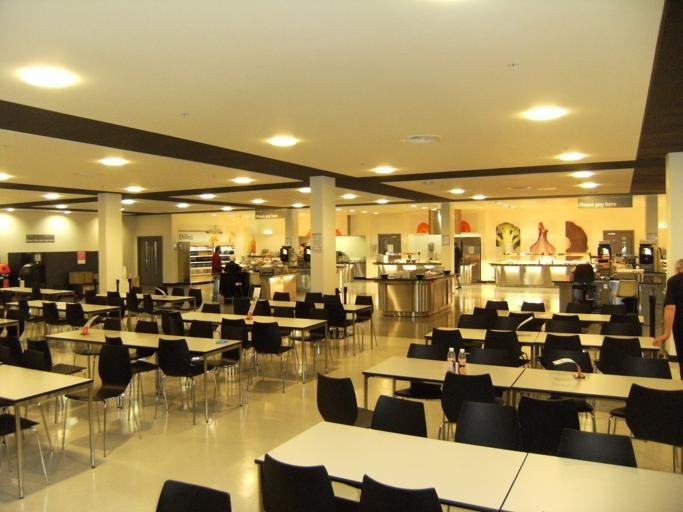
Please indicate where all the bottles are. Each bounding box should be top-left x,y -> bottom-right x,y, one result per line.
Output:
446,347 -> 467,376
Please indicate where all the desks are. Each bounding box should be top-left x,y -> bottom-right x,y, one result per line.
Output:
490,258 -> 588,287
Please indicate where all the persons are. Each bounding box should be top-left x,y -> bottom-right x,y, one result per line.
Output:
454,243 -> 463,290
210,245 -> 224,301
652,258 -> 683,381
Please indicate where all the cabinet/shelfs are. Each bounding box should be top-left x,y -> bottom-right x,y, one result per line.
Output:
190,246 -> 234,284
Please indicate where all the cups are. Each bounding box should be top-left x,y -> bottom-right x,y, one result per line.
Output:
79,326 -> 89,335
